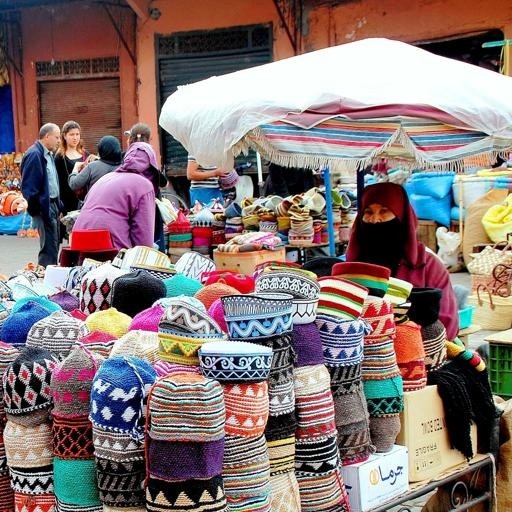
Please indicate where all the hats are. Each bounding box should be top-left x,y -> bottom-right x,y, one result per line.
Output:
0,152 -> 38,237
131,124 -> 150,136
168,188 -> 358,262
218,169 -> 239,188
0,230 -> 485,512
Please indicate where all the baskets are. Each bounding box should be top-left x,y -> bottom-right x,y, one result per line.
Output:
489,342 -> 512,396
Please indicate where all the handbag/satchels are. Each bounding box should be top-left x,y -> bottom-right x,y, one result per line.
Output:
466,240 -> 512,330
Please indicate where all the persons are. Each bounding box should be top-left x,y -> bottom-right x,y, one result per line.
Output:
187,152 -> 229,208
55,121 -> 91,249
124,124 -> 165,256
68,135 -> 126,195
345,183 -> 459,342
21,123 -> 63,267
71,142 -> 156,252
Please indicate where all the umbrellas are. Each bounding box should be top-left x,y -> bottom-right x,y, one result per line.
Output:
158,38 -> 512,256
